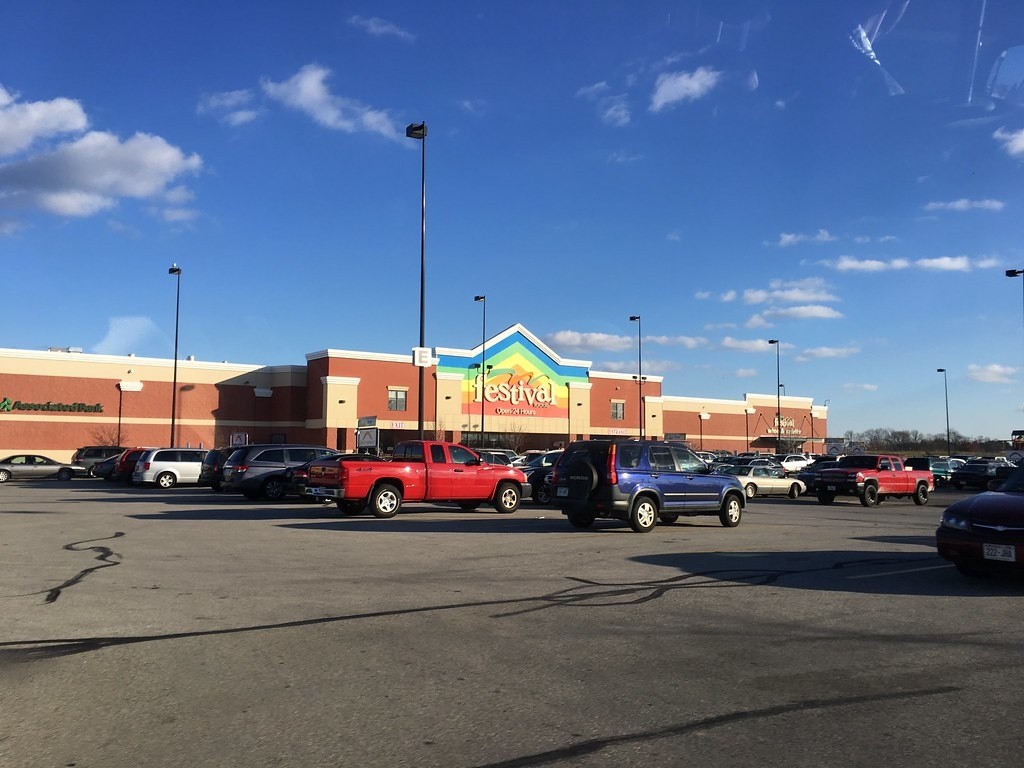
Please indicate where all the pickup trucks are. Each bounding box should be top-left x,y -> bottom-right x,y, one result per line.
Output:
813,454 -> 935,507
303,442 -> 533,519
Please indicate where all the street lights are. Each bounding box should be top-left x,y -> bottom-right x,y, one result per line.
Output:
167,264 -> 182,448
404,118 -> 430,442
936,368 -> 952,456
473,293 -> 485,448
630,315 -> 644,438
768,337 -> 783,454
779,383 -> 785,396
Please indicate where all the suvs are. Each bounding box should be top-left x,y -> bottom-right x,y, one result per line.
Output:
71,446 -> 125,468
546,438 -> 747,534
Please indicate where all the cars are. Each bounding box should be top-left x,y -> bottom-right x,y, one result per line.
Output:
776,454 -> 816,472
692,449 -> 783,475
196,445 -> 238,493
281,452 -> 388,503
902,455 -> 1024,491
219,443 -> 341,501
92,454 -> 121,481
722,465 -> 806,499
113,448 -> 147,477
936,460 -> 1024,579
471,447 -> 565,505
0,455 -> 89,485
131,448 -> 209,489
